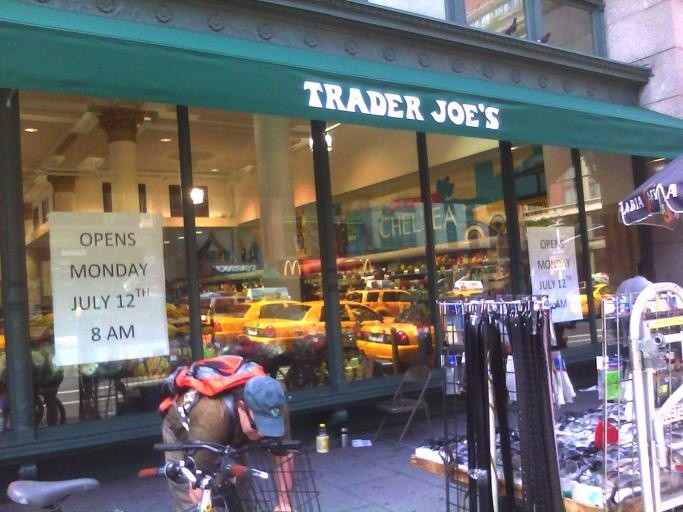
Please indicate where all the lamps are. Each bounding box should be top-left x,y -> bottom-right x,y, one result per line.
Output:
308,123 -> 340,152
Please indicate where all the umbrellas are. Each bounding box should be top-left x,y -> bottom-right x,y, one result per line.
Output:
616,153 -> 682,231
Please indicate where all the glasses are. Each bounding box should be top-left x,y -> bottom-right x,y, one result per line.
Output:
244,408 -> 258,430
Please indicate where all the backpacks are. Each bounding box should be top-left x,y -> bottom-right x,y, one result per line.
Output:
157,352 -> 268,400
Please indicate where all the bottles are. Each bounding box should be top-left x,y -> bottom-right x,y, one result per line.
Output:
315,423 -> 330,453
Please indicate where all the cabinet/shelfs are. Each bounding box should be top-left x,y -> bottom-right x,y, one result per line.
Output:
174,234 -> 514,305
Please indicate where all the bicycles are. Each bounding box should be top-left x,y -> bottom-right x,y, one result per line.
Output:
5,438 -> 322,511
0,384 -> 67,432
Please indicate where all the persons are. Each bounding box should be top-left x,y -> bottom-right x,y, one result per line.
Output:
613,257 -> 653,371
161,354 -> 299,511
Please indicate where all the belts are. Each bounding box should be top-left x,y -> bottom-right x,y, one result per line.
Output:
466,297 -> 567,512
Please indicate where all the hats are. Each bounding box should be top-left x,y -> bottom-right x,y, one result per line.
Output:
242,375 -> 287,439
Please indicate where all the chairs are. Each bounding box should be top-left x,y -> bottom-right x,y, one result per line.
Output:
371,364 -> 436,444
